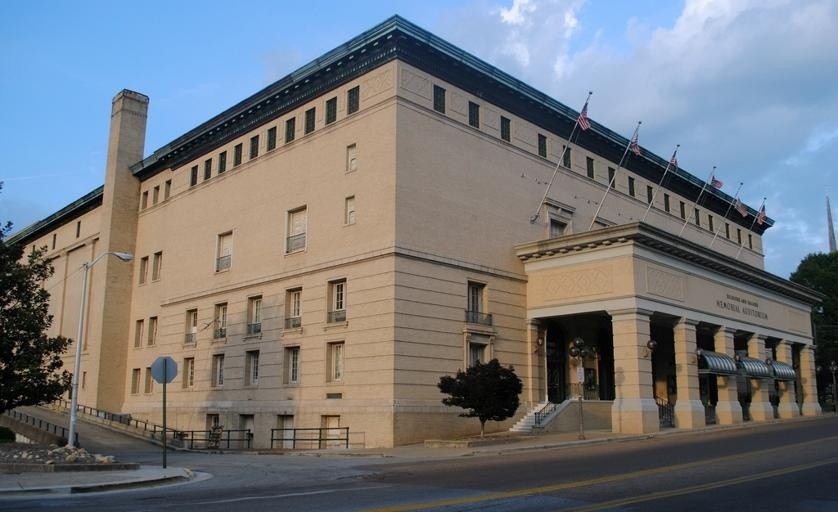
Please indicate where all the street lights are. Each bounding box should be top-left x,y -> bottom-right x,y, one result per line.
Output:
569,337 -> 591,440
66,250 -> 135,452
